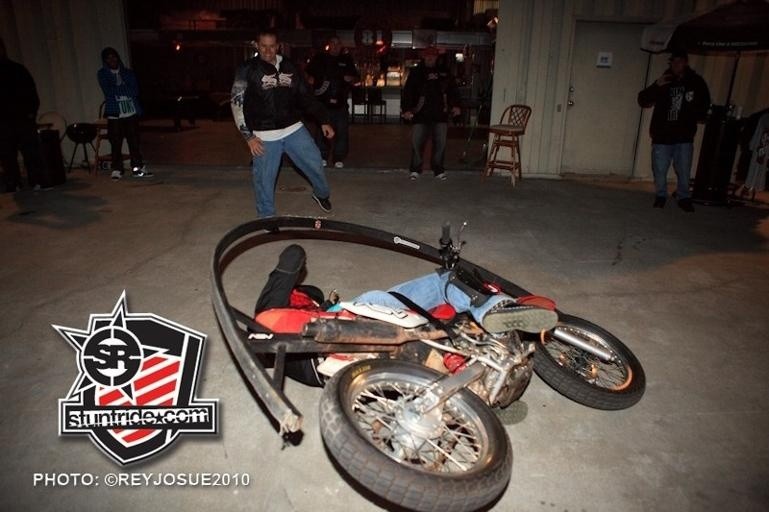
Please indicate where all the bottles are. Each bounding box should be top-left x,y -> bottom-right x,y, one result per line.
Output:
724,101 -> 744,120
442,352 -> 466,374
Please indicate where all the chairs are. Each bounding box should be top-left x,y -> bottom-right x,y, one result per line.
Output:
92,100 -> 136,174
481,104 -> 532,186
350,88 -> 370,120
368,88 -> 386,120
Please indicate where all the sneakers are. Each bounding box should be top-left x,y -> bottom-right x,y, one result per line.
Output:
131,166 -> 154,179
434,172 -> 449,183
652,192 -> 669,212
110,170 -> 124,180
409,171 -> 422,184
673,192 -> 696,214
311,191 -> 333,215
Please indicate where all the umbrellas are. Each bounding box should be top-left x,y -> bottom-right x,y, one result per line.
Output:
643,0 -> 769,184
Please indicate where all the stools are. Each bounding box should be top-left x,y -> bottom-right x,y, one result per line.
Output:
66,123 -> 96,174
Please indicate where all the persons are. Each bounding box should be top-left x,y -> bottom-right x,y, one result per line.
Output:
304,35 -> 353,169
229,26 -> 336,218
97,47 -> 154,181
638,49 -> 711,211
400,46 -> 463,181
0,40 -> 55,192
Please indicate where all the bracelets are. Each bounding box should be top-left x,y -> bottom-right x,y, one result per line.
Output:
247,135 -> 255,142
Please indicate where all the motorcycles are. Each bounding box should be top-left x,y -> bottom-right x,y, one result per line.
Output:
208,212 -> 647,511
